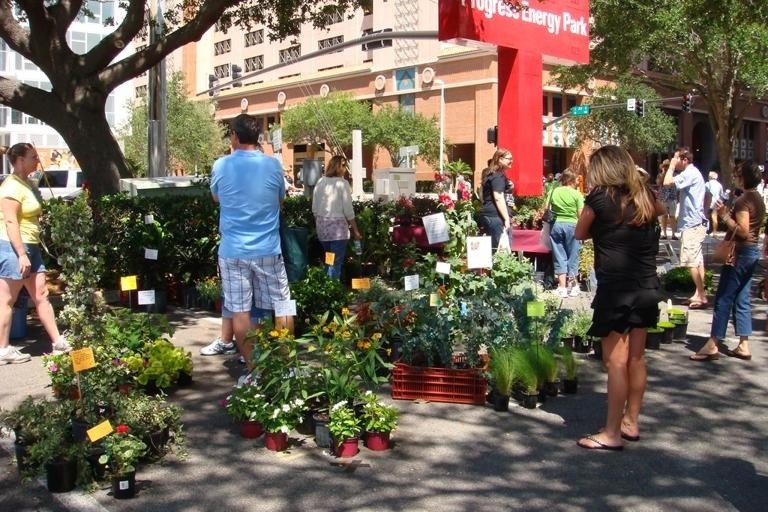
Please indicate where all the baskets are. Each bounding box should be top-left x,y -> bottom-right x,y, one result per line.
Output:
391,352 -> 490,407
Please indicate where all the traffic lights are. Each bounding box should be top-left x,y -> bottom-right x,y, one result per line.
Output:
235,66 -> 242,87
212,75 -> 218,95
681,93 -> 690,112
636,99 -> 644,118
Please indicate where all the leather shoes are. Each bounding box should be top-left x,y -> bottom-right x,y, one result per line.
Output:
672,236 -> 678,240
660,236 -> 667,239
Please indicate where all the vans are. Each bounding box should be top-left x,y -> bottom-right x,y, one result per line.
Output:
36,166 -> 84,202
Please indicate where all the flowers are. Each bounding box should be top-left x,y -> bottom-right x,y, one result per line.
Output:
0,159 -> 602,503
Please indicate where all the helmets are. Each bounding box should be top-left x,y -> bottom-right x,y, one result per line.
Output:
659,159 -> 670,170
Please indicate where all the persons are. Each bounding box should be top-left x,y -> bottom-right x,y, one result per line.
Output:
0,140 -> 73,368
475,144 -> 766,360
573,144 -> 666,449
200,116 -> 363,393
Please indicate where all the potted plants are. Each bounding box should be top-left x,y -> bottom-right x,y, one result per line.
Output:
645,265 -> 716,351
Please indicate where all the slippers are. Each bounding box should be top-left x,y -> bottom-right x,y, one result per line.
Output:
682,298 -> 693,305
689,353 -> 719,361
689,300 -> 708,310
729,350 -> 751,361
578,435 -> 624,451
598,425 -> 640,440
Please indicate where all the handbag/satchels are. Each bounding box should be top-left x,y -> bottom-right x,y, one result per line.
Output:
542,209 -> 557,223
280,226 -> 309,283
711,226 -> 738,268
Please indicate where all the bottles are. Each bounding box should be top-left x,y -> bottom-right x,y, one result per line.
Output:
354,238 -> 362,256
713,189 -> 730,212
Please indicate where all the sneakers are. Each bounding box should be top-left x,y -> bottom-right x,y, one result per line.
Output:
0,345 -> 31,364
237,374 -> 261,389
547,283 -> 568,298
52,336 -> 73,355
202,337 -> 236,355
570,285 -> 581,297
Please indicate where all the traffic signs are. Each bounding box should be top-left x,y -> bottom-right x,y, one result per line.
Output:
627,98 -> 636,111
571,105 -> 590,115
